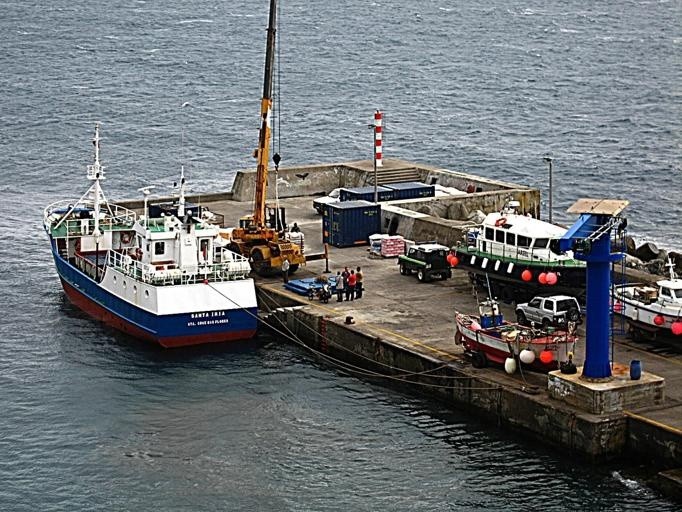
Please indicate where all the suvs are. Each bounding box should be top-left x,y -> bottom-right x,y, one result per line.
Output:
396,244 -> 452,283
515,294 -> 583,328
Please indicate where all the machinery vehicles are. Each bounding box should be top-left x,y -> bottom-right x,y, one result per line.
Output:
218,0 -> 331,279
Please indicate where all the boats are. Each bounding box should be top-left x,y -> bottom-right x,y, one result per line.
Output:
455,273 -> 579,364
451,201 -> 614,279
609,258 -> 682,332
42,123 -> 259,349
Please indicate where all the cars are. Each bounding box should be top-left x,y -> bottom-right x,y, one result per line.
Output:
312,187 -> 349,215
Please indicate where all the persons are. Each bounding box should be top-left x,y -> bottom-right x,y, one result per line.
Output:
291,223 -> 301,231
333,269 -> 344,303
343,270 -> 356,301
340,267 -> 350,287
281,255 -> 290,282
354,267 -> 365,299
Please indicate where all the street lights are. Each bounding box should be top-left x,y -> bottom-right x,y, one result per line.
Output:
542,156 -> 553,224
